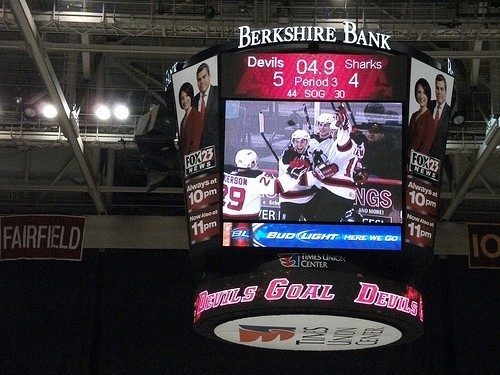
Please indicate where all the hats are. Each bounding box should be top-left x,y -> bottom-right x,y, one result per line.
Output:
367,122 -> 385,133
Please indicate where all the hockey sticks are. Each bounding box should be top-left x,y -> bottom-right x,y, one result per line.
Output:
259,112 -> 280,162
303,102 -> 326,172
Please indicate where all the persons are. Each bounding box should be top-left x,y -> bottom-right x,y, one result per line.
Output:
223,149 -> 311,219
279,106 -> 401,222
428,74 -> 451,161
193,63 -> 219,149
408,78 -> 436,155
179,82 -> 203,155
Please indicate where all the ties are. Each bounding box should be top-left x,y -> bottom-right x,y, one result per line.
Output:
201,94 -> 207,113
434,105 -> 441,122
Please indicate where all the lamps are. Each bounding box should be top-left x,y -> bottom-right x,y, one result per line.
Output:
452,105 -> 467,126
24,91 -> 45,120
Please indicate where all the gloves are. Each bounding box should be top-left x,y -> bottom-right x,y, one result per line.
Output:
286,157 -> 311,180
312,163 -> 339,182
334,105 -> 350,131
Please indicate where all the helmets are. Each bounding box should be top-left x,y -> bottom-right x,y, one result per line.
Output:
290,129 -> 310,155
317,113 -> 334,126
234,149 -> 259,170
330,120 -> 352,134
